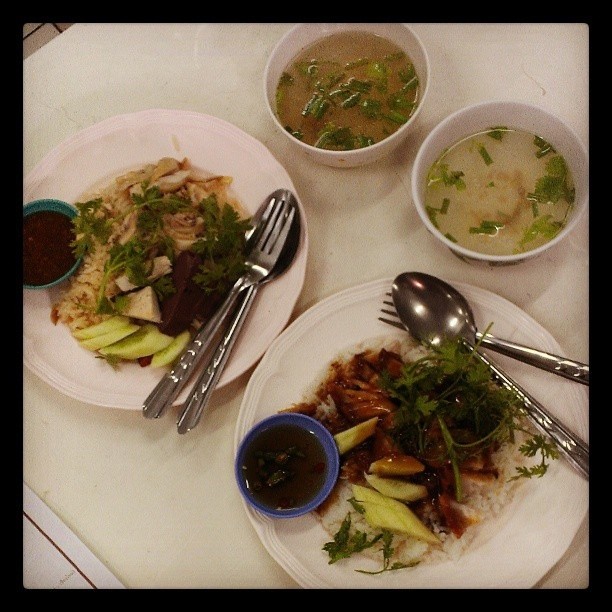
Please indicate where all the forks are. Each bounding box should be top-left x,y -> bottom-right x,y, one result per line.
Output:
378,292 -> 589,387
142,196 -> 296,421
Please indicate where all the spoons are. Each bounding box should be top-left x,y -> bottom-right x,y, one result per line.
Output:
176,187 -> 301,435
391,271 -> 589,482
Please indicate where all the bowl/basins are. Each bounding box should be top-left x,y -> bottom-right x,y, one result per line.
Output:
262,23 -> 431,170
410,100 -> 589,269
234,413 -> 341,519
23,198 -> 87,290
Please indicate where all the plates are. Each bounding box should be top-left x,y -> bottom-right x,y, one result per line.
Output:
22,108 -> 310,412
233,276 -> 590,589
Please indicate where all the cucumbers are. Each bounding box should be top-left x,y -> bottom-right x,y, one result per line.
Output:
331,417 -> 443,550
73,316 -> 190,369
